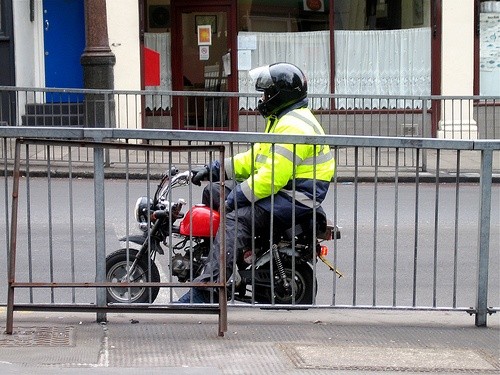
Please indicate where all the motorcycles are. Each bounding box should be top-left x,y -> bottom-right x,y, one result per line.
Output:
105,166 -> 344,311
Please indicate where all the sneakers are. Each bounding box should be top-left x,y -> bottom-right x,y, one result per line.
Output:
173,287 -> 220,304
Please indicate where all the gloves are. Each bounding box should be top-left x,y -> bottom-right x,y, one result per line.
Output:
218,200 -> 232,214
192,166 -> 209,186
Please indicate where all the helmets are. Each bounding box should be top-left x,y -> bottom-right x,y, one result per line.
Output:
249,62 -> 308,119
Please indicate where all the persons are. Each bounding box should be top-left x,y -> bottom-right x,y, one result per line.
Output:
178,61 -> 335,304
